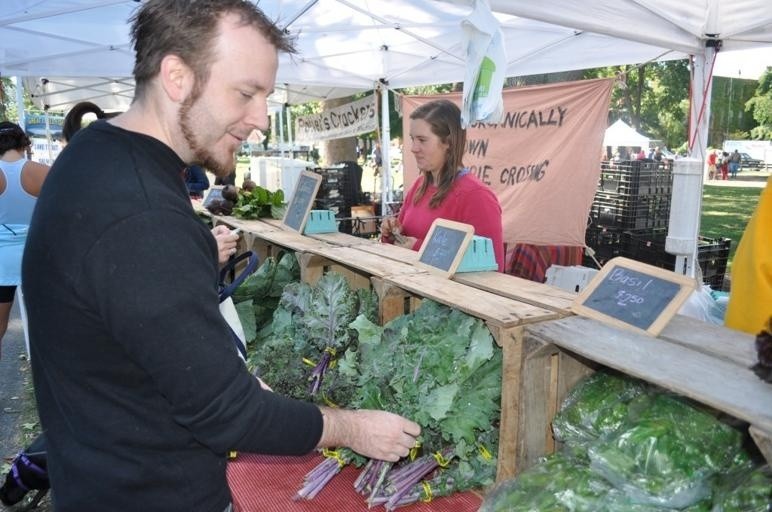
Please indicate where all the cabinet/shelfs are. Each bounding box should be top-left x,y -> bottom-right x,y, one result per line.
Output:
191,188 -> 769,512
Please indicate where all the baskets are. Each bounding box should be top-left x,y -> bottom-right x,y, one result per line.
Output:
306,163 -> 357,234
589,159 -> 731,290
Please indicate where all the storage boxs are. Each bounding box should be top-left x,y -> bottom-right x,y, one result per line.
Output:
307,160 -> 363,230
582,159 -> 731,291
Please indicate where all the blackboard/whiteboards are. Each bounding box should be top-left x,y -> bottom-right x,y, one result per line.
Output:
284,169 -> 323,234
570,256 -> 698,339
200,185 -> 226,207
413,218 -> 475,280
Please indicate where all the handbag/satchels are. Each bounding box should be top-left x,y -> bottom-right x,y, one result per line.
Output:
217,251 -> 259,364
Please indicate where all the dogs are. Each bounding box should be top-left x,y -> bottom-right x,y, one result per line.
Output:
0,432 -> 51,512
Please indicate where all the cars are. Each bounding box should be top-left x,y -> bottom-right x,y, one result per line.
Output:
726,152 -> 766,171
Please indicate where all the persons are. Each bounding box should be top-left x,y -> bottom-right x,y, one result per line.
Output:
61,101 -> 241,266
721,152 -> 729,179
382,98 -> 504,273
708,150 -> 718,180
682,173 -> 771,427
183,166 -> 209,200
609,146 -> 668,169
19,0 -> 420,512
0,120 -> 52,361
731,149 -> 741,177
